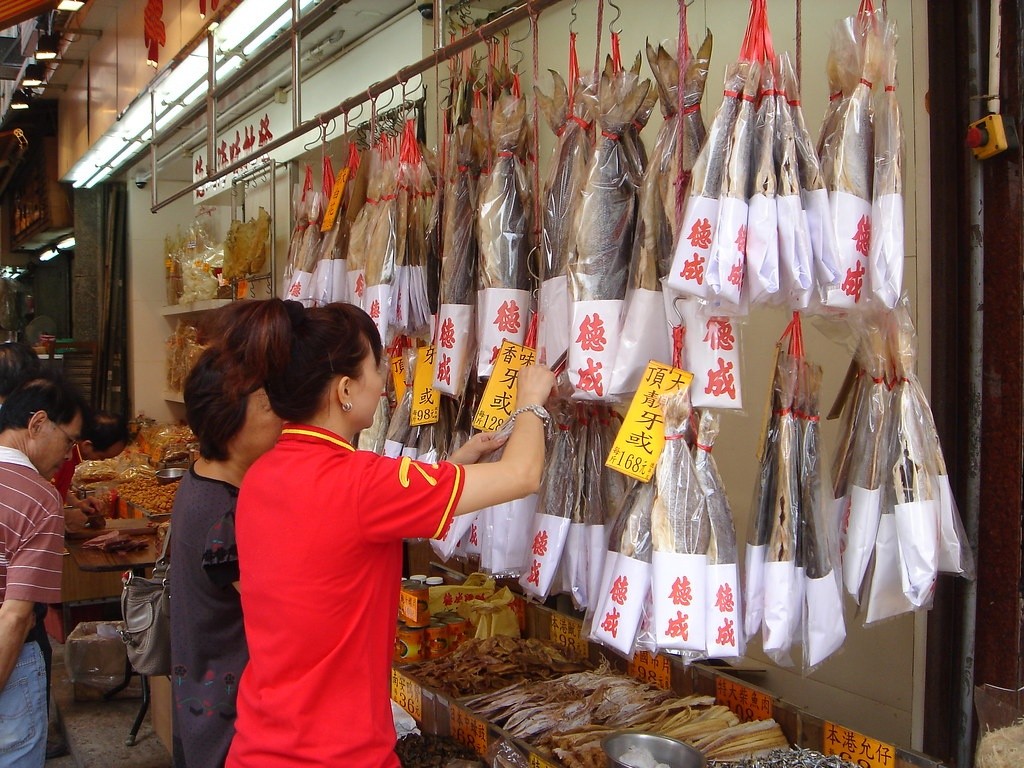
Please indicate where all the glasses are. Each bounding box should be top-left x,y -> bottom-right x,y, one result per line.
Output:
30,410 -> 78,454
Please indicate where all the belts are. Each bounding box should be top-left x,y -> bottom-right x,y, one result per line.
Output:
23,629 -> 37,643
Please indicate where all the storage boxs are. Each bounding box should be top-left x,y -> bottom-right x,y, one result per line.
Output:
66,621 -> 143,703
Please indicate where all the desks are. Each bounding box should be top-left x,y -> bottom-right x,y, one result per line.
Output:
66,517 -> 171,747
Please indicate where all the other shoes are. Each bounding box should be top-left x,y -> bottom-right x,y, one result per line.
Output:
46,741 -> 67,759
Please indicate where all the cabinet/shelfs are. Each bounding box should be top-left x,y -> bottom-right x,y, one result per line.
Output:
162,298 -> 233,404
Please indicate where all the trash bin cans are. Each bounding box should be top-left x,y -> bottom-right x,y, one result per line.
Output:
67,620 -> 127,701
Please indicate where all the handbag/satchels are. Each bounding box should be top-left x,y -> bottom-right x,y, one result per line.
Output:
120,522 -> 172,677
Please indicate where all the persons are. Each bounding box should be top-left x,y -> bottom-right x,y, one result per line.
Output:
224,301 -> 559,768
171,347 -> 283,768
0,341 -> 129,768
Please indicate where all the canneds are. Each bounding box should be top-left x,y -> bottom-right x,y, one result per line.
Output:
394,574 -> 468,665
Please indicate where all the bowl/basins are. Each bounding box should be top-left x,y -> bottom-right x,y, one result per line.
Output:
600,730 -> 707,768
155,468 -> 188,486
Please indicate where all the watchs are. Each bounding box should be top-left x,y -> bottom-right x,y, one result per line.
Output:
512,405 -> 552,428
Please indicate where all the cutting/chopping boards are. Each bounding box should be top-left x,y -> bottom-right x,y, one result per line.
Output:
65,517 -> 155,541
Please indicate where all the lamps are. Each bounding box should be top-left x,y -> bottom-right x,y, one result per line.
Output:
56,0 -> 88,11
10,83 -> 67,110
34,27 -> 102,60
22,58 -> 84,87
